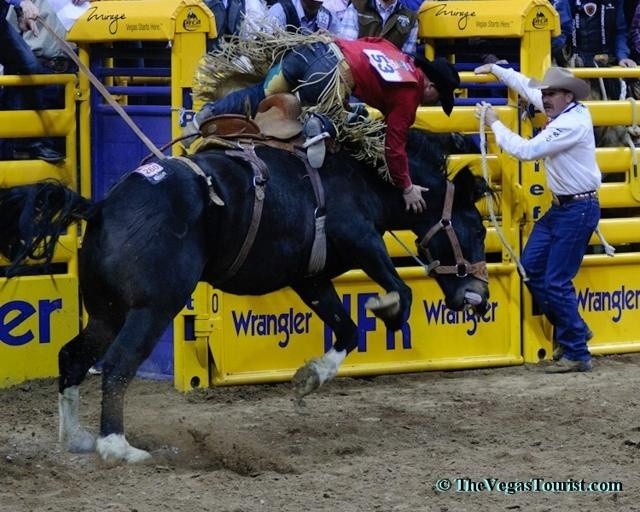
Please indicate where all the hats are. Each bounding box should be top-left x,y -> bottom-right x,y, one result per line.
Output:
414,53 -> 461,118
528,63 -> 592,102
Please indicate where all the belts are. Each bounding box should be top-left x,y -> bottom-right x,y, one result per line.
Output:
319,33 -> 354,90
548,190 -> 598,206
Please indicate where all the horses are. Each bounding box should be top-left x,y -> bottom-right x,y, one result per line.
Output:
0,126 -> 503,466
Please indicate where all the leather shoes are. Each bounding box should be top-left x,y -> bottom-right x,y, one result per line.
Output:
12,139 -> 66,163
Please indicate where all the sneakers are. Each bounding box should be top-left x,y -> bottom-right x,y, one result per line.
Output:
552,327 -> 594,359
179,107 -> 210,152
544,355 -> 593,374
304,113 -> 325,171
